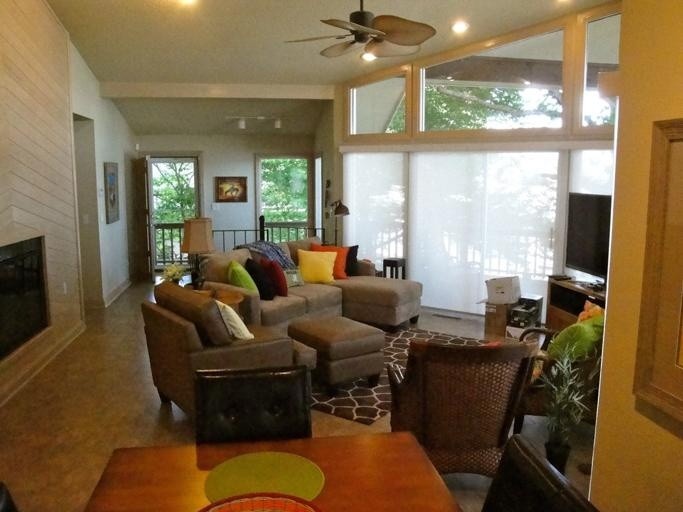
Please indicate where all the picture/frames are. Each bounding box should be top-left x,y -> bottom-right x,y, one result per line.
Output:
215,177 -> 247,203
104,162 -> 119,224
632,119 -> 683,427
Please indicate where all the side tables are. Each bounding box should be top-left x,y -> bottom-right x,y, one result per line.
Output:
184,284 -> 244,321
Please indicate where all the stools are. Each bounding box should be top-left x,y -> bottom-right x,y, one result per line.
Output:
384,258 -> 405,280
288,316 -> 385,397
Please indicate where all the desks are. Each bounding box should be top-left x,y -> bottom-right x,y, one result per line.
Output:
543,278 -> 606,329
84,432 -> 463,512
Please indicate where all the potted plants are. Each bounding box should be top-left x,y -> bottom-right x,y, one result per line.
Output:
530,341 -> 591,475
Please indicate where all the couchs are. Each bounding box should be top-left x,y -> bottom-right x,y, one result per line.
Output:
198,236 -> 423,334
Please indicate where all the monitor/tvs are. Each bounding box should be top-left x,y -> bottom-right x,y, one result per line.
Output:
565,192 -> 612,291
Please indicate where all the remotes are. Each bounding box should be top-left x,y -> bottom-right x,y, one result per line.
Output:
554,276 -> 571,281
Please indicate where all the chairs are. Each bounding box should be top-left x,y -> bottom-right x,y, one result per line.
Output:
142,282 -> 317,422
195,365 -> 312,444
387,338 -> 539,479
481,433 -> 600,512
513,327 -> 602,434
0,482 -> 17,512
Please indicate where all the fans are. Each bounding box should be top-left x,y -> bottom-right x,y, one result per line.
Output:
285,0 -> 435,58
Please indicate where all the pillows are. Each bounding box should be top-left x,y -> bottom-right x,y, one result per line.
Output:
213,297 -> 255,339
228,243 -> 359,300
154,283 -> 232,346
547,317 -> 603,360
577,299 -> 605,322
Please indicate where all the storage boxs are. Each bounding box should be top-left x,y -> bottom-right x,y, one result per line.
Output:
486,276 -> 521,303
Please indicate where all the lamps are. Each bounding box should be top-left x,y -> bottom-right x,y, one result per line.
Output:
226,116 -> 287,130
181,218 -> 216,289
333,200 -> 349,246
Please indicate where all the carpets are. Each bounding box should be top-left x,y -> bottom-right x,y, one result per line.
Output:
311,325 -> 490,425
